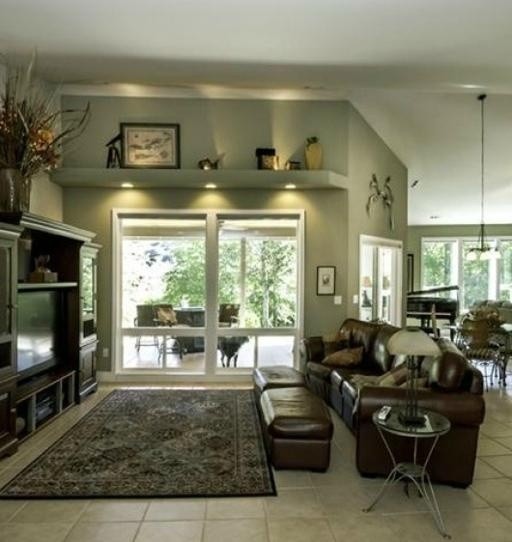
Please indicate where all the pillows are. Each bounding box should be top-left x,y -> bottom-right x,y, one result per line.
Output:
351,360 -> 412,386
398,376 -> 428,388
321,345 -> 364,366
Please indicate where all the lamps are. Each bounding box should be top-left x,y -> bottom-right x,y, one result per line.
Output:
466,94 -> 501,261
387,328 -> 443,427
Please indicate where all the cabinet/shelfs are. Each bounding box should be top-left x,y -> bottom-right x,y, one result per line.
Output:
0,210 -> 97,447
0,222 -> 25,459
80,241 -> 102,399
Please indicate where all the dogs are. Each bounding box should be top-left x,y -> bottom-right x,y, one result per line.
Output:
216,336 -> 250,368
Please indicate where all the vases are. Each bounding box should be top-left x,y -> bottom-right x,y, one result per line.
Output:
0,168 -> 30,212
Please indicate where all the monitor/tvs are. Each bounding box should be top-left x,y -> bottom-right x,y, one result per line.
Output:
17,289 -> 65,381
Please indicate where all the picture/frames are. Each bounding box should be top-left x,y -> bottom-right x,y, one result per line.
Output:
407,254 -> 414,293
119,121 -> 180,168
316,266 -> 336,296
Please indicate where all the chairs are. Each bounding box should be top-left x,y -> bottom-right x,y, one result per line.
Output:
133,303 -> 242,363
445,298 -> 512,391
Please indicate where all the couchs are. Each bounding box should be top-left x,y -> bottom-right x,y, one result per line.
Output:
299,318 -> 485,490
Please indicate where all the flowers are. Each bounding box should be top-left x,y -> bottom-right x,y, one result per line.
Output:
0,65 -> 95,177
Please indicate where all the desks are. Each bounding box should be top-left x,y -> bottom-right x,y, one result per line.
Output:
362,406 -> 451,539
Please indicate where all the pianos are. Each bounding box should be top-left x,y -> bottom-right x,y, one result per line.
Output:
407,285 -> 459,340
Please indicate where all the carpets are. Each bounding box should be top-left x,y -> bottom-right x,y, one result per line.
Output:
0,389 -> 278,501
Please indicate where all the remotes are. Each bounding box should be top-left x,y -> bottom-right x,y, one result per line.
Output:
378,405 -> 392,421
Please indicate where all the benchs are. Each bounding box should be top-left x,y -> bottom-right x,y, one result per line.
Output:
252,364 -> 307,401
260,386 -> 333,472
